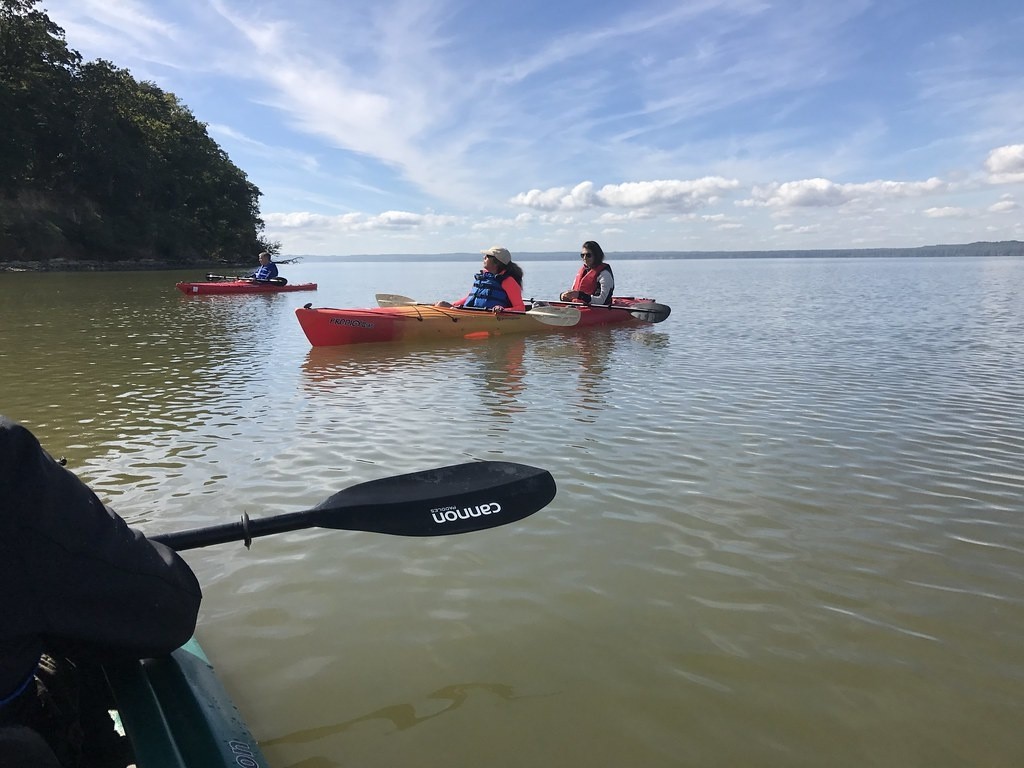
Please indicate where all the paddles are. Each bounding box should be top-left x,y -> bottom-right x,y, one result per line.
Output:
523,298 -> 672,323
206,272 -> 287,286
375,293 -> 581,327
149,460 -> 557,603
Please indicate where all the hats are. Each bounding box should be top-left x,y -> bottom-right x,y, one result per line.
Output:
481,246 -> 511,265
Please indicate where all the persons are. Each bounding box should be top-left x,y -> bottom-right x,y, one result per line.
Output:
560,241 -> 615,308
238,252 -> 278,282
0,417 -> 203,768
435,246 -> 525,314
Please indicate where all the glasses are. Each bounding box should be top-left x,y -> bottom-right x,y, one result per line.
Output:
486,255 -> 491,258
581,253 -> 592,258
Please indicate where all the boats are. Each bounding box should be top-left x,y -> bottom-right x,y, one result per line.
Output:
294,295 -> 657,347
0,623 -> 270,768
175,279 -> 318,296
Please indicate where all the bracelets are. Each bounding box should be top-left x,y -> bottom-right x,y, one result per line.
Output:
560,293 -> 563,301
